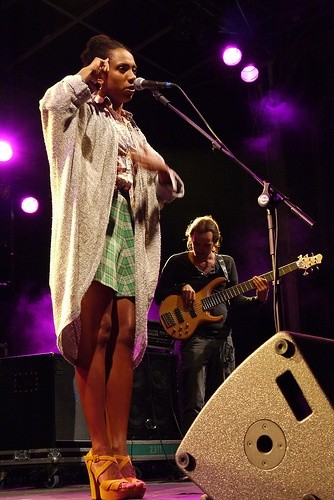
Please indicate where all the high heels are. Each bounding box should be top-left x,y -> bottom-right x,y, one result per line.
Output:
83,450 -> 133,500
114,453 -> 146,500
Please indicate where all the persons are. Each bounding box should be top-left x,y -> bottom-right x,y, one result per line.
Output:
39,35 -> 184,500
154,216 -> 269,422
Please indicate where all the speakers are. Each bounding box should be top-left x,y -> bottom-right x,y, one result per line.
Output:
125,353 -> 176,441
175,331 -> 333,500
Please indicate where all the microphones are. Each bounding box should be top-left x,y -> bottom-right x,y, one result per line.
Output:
133,77 -> 177,92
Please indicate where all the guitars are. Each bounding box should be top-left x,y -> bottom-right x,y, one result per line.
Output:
158,251 -> 323,341
